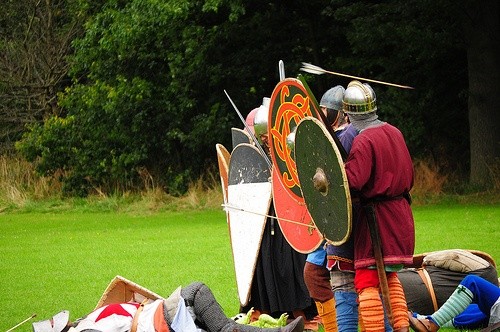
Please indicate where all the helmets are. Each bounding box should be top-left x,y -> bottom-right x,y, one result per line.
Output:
31,310 -> 69,332
342,80 -> 377,115
243,106 -> 259,135
252,96 -> 273,145
319,84 -> 344,110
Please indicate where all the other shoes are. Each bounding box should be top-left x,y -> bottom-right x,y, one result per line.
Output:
408,310 -> 439,332
280,316 -> 305,332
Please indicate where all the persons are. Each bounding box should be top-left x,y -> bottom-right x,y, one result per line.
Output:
407,273 -> 500,332
32,281 -> 305,332
244,79 -> 416,332
